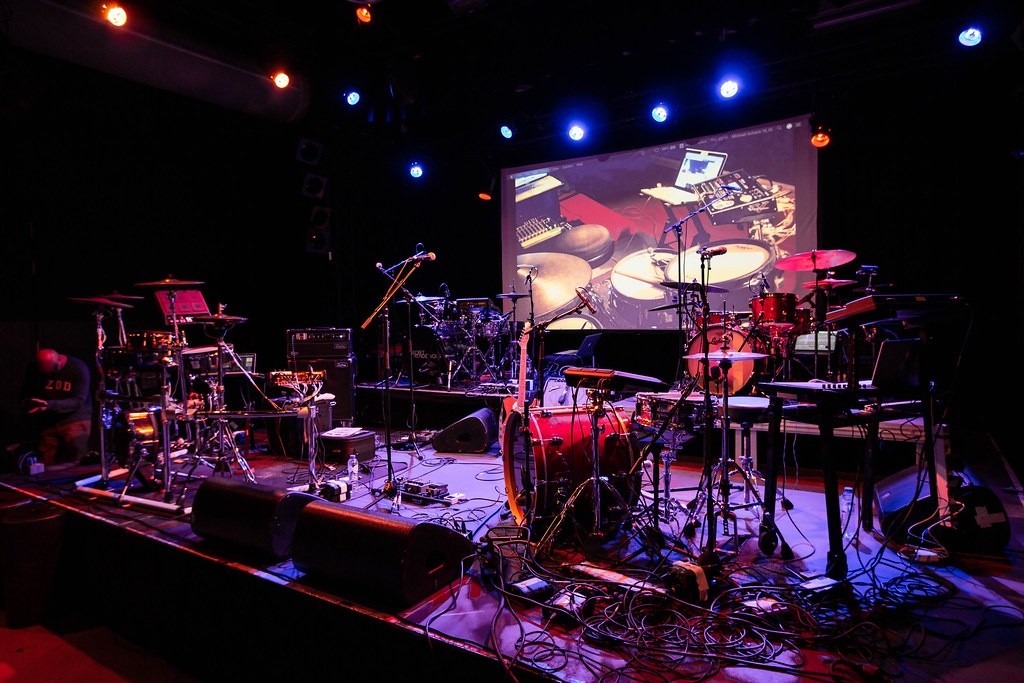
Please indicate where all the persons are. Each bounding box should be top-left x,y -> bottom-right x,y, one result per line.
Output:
16,348 -> 92,467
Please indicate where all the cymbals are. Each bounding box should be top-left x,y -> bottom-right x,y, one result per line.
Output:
496,291 -> 530,300
775,249 -> 857,272
192,313 -> 249,325
682,350 -> 771,360
72,296 -> 135,309
802,278 -> 857,289
395,296 -> 445,304
134,278 -> 205,287
660,282 -> 730,293
101,293 -> 145,300
648,301 -> 700,311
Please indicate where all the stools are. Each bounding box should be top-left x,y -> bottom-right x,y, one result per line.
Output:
553,223 -> 616,269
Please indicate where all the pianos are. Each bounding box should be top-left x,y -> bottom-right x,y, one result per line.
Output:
823,293 -> 965,329
516,174 -> 574,250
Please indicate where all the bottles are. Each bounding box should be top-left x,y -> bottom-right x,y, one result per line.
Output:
839,486 -> 856,538
348,455 -> 358,492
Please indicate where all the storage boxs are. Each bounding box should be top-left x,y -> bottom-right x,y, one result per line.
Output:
289,356 -> 358,428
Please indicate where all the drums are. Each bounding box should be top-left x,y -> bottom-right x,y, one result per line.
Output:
482,320 -> 509,337
180,342 -> 235,374
103,345 -> 165,404
694,309 -> 736,334
765,307 -> 812,338
126,331 -> 181,361
545,315 -> 604,330
609,246 -> 680,329
749,291 -> 800,328
435,320 -> 464,341
515,252 -> 592,321
663,237 -> 777,315
113,407 -> 159,469
634,391 -> 719,430
501,403 -> 643,545
469,307 -> 500,325
685,325 -> 768,396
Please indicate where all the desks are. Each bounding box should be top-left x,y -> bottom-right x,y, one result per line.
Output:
716,416 -> 950,527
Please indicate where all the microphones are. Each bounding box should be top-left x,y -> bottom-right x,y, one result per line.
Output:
515,494 -> 526,506
411,253 -> 437,261
524,269 -> 533,284
375,262 -> 383,269
576,290 -> 596,314
704,247 -> 727,258
443,285 -> 450,297
722,185 -> 742,192
711,366 -> 721,394
762,274 -> 770,288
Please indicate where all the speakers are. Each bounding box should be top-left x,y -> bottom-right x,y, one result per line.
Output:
290,357 -> 355,423
432,408 -> 498,454
292,501 -> 477,612
872,463 -> 967,548
192,478 -> 328,560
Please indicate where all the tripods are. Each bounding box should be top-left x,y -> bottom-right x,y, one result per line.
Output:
113,286 -> 259,504
361,258 -> 815,572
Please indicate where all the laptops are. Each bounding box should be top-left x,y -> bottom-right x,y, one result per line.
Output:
640,148 -> 729,205
860,336 -> 933,389
